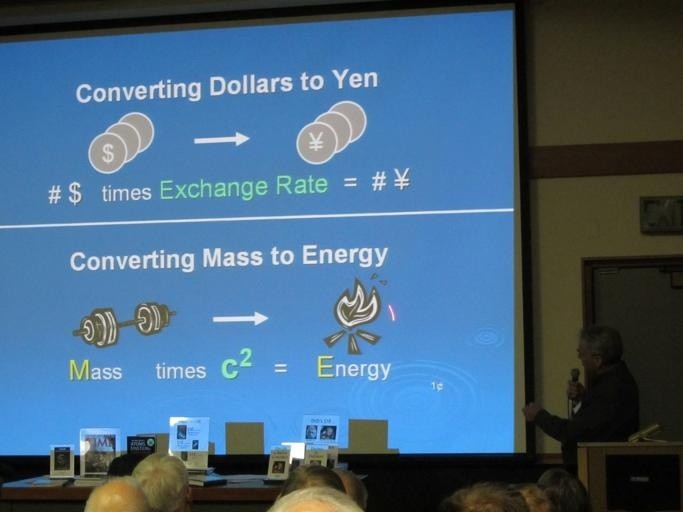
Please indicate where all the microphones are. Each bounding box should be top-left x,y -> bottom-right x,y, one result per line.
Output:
570,367 -> 581,385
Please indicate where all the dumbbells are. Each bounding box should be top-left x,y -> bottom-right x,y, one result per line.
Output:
73,303 -> 177,348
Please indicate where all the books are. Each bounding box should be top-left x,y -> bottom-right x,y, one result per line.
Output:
31,476 -> 69,487
189,473 -> 227,487
74,473 -> 107,487
263,476 -> 288,486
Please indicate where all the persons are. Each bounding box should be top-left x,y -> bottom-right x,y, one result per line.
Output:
508,481 -> 553,511
83,475 -> 151,512
178,427 -> 185,439
322,427 -> 333,439
330,467 -> 368,511
438,480 -> 530,512
275,463 -> 347,503
521,322 -> 640,479
131,451 -> 189,511
537,467 -> 588,511
306,427 -> 317,439
266,484 -> 363,512
